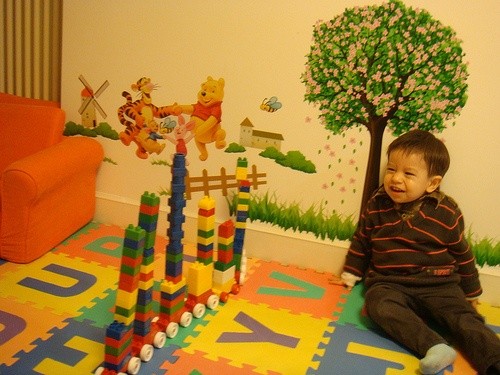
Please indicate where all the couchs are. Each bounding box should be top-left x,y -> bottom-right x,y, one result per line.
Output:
0,92 -> 104,264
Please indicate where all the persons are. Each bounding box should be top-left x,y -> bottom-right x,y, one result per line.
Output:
327,130 -> 500,375
